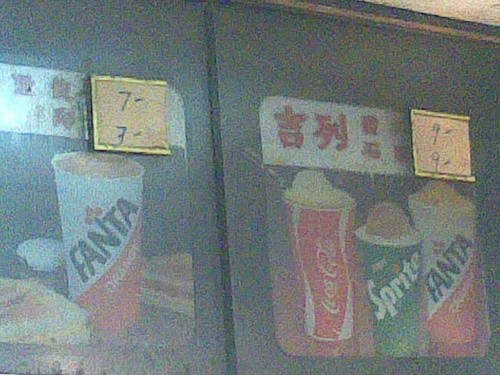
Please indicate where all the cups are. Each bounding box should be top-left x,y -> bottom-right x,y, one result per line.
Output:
353,226 -> 422,358
51,150 -> 144,327
407,196 -> 489,358
282,186 -> 358,342
